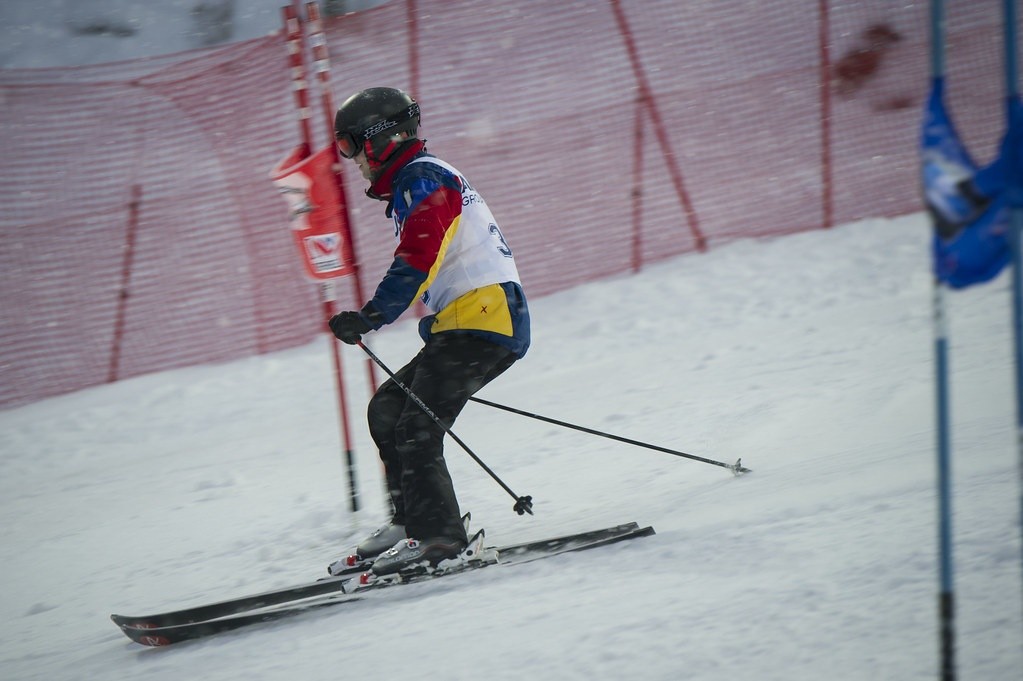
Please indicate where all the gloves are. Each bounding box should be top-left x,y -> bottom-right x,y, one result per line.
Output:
329,311 -> 373,345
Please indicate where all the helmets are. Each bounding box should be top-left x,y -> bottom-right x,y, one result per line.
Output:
334,87 -> 420,164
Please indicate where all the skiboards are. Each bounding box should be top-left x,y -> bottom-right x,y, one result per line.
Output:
108,521 -> 657,647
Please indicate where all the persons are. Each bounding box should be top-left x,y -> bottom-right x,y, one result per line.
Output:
328,87 -> 532,594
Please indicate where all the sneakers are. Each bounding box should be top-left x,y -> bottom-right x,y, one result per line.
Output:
356,522 -> 406,558
373,538 -> 466,576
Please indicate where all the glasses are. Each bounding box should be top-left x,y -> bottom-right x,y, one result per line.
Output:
334,130 -> 364,159
